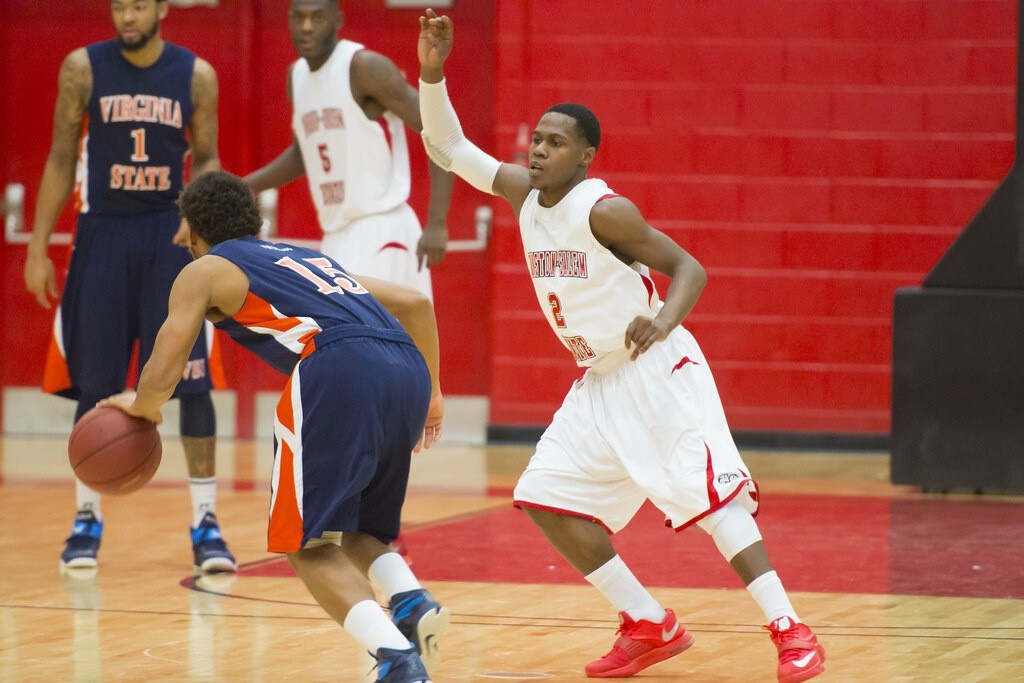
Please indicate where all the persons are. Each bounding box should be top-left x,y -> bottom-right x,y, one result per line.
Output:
238,0 -> 450,302
414,8 -> 827,683
23,1 -> 240,576
96,166 -> 448,682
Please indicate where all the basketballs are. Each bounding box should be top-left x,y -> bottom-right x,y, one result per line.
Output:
66,405 -> 163,495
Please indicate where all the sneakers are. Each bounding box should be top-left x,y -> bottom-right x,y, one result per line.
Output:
60,509 -> 103,568
585,607 -> 695,678
762,616 -> 826,683
381,589 -> 449,680
367,642 -> 432,683
189,511 -> 237,572
